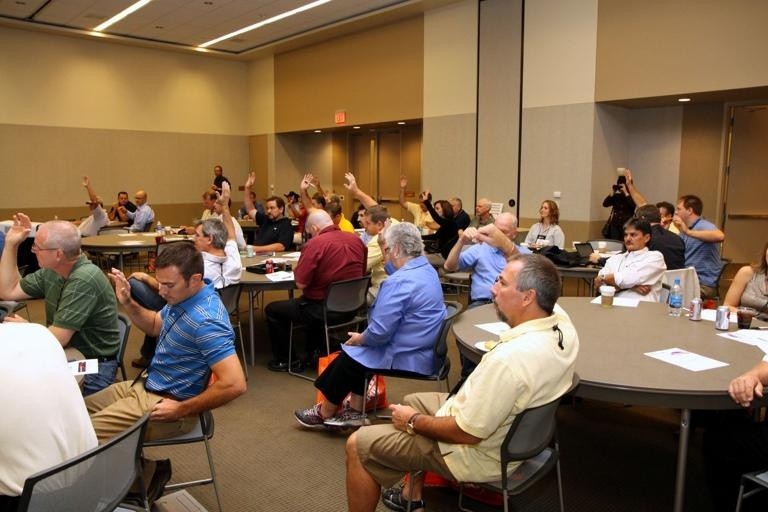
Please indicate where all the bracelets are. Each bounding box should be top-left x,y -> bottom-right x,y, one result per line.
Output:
501,239 -> 517,261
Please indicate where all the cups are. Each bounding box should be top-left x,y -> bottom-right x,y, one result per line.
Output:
247,245 -> 253,258
736,307 -> 754,329
155,235 -> 163,244
600,286 -> 615,307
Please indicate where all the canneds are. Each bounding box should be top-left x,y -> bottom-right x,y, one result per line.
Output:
265,259 -> 273,274
246,245 -> 254,257
715,305 -> 731,331
689,299 -> 703,321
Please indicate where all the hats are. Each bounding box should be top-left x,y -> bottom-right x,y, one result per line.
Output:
86,196 -> 103,205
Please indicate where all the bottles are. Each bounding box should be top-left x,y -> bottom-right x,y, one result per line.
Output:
156,220 -> 161,233
162,230 -> 166,242
669,278 -> 683,317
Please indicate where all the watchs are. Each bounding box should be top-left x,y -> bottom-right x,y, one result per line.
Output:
401,411 -> 425,436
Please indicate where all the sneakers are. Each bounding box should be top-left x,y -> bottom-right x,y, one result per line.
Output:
293,403 -> 352,434
323,400 -> 371,427
381,487 -> 425,512
132,356 -> 148,368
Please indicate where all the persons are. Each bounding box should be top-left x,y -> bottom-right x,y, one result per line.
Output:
0,304 -> 104,511
82,240 -> 250,508
288,221 -> 449,437
594,168 -> 725,304
722,246 -> 767,313
400,174 -> 565,308
243,170 -> 400,372
1,175 -> 155,396
124,165 -> 245,369
343,222 -> 581,512
728,355 -> 766,407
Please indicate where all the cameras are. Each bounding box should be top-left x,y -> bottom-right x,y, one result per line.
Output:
613,185 -> 622,192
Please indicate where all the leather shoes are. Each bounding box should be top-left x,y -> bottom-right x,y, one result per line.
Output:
123,459 -> 172,510
267,357 -> 304,373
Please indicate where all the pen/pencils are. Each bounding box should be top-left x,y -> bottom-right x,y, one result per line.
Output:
750,326 -> 768,329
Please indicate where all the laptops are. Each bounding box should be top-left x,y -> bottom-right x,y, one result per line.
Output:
575,244 -> 594,257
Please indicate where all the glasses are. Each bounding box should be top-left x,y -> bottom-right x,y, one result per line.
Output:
31,243 -> 59,252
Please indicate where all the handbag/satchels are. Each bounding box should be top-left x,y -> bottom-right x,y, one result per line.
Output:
315,351 -> 389,412
601,215 -> 621,240
534,245 -> 581,268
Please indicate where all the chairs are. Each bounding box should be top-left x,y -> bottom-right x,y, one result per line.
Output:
360,300 -> 464,420
736,468 -> 767,511
136,365 -> 225,511
214,283 -> 249,381
4,300 -> 31,322
112,313 -> 132,380
700,258 -> 732,304
467,274 -> 474,305
283,267 -> 374,382
13,409 -> 156,512
0,213 -> 744,291
410,374 -> 580,512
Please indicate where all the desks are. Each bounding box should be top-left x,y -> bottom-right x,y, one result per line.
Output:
453,295 -> 767,512
219,249 -> 307,370
64,345 -> 89,391
